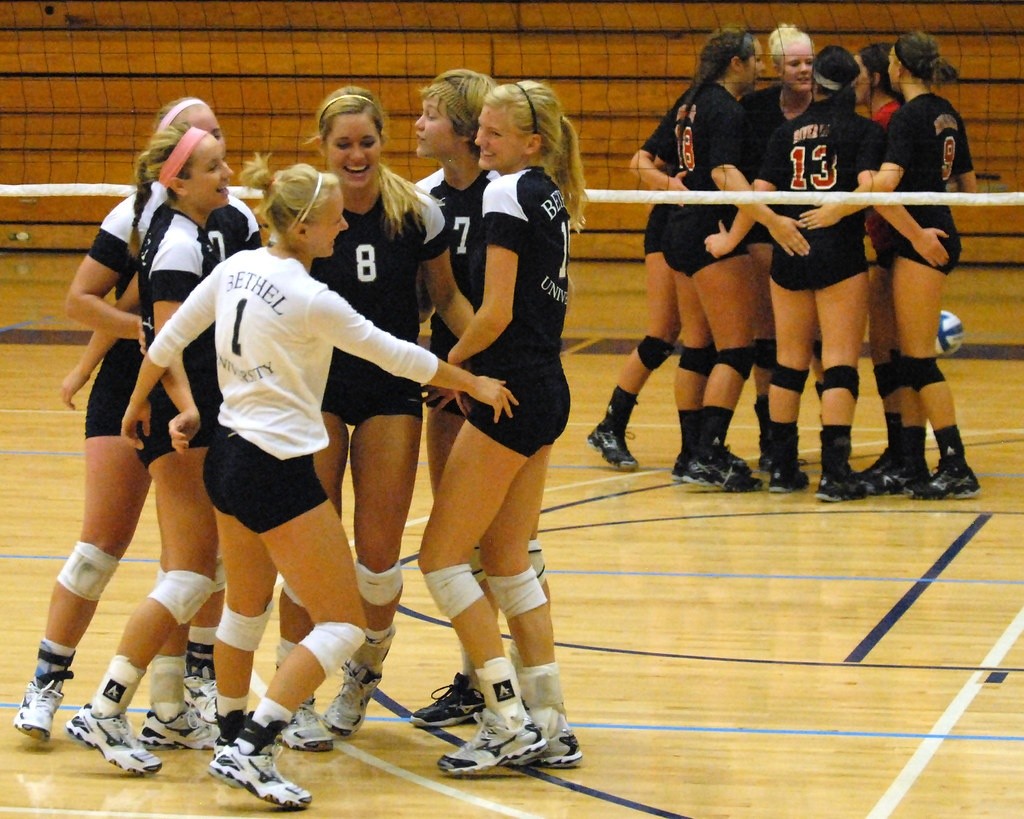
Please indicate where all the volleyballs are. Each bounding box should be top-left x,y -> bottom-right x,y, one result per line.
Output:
934,309 -> 964,357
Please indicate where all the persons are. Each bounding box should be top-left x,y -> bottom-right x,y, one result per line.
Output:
587,25 -> 981,501
14,70 -> 587,809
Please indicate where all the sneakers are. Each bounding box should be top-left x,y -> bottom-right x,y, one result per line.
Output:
529,729 -> 583,768
13,679 -> 64,742
758,453 -> 808,469
437,708 -> 548,775
873,461 -> 930,495
183,675 -> 218,723
862,448 -> 898,477
280,698 -> 335,752
768,466 -> 809,493
683,457 -> 764,492
721,445 -> 748,465
410,671 -> 486,728
814,473 -> 873,501
321,658 -> 382,735
213,734 -> 234,760
587,425 -> 639,469
67,704 -> 163,775
207,742 -> 313,809
903,459 -> 981,501
136,710 -> 220,750
670,451 -> 752,481
